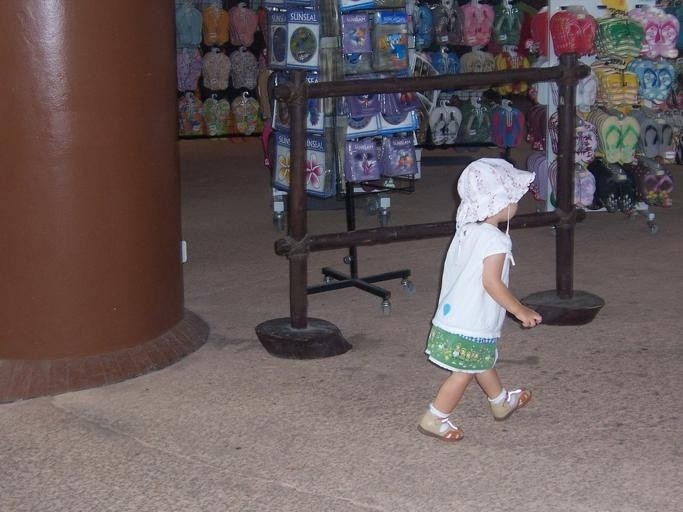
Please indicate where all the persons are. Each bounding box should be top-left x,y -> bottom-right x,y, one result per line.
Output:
416,158 -> 542,443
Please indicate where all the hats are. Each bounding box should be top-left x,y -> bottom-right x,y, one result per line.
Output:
455,157 -> 536,226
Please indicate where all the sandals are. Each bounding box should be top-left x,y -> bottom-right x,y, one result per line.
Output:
491,388 -> 532,420
416,410 -> 464,442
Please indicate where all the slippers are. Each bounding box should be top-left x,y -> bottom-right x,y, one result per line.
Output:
176,2 -> 259,138
412,1 -> 531,149
526,4 -> 682,215
